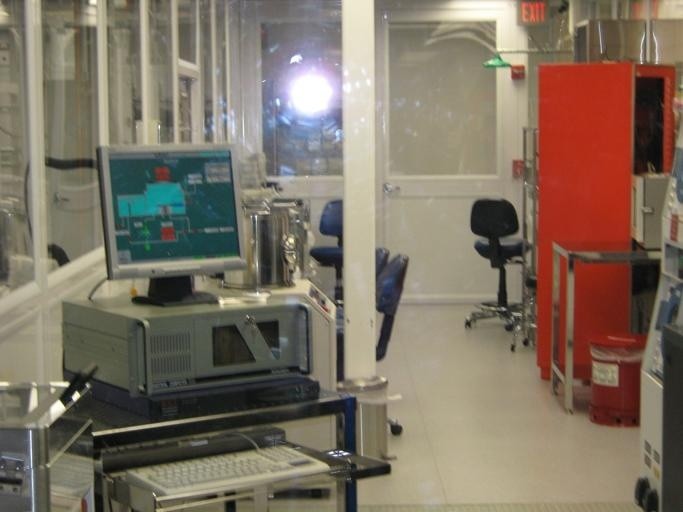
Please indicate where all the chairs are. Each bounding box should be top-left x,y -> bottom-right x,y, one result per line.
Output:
464,197 -> 532,333
308,200 -> 409,436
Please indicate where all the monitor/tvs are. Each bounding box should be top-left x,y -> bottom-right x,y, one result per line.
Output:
96,143 -> 248,307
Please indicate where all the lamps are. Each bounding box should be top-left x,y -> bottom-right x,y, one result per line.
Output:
483,48 -> 573,68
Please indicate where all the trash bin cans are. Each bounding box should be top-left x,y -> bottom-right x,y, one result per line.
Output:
336,377 -> 397,462
588,333 -> 649,427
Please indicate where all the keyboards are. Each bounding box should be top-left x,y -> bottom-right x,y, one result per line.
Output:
126,445 -> 331,496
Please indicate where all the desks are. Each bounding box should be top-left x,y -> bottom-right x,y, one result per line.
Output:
69,393 -> 391,510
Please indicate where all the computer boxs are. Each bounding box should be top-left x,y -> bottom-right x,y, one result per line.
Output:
62,291 -> 319,423
